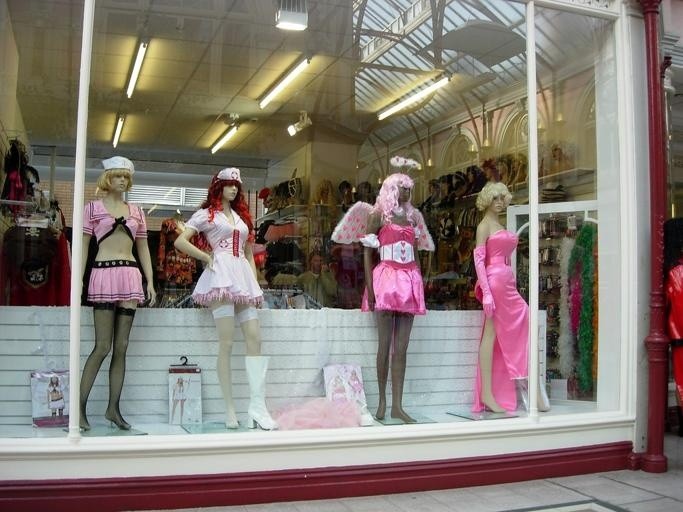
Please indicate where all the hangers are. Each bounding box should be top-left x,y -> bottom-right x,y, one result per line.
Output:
162,286 -> 319,309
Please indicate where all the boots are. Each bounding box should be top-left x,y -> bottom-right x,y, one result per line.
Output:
245,356 -> 278,429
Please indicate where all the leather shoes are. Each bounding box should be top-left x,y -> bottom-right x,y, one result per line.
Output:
355,397 -> 375,426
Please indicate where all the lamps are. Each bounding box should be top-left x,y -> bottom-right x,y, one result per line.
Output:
211,122 -> 238,154
113,113 -> 126,149
377,71 -> 451,120
258,51 -> 312,109
126,38 -> 149,99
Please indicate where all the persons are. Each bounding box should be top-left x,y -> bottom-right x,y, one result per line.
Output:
296,250 -> 338,308
330,154 -> 436,423
169,375 -> 187,424
46,376 -> 65,420
426,139 -> 575,201
331,376 -> 346,401
347,369 -> 363,395
173,166 -> 279,431
79,154 -> 157,430
469,180 -> 551,415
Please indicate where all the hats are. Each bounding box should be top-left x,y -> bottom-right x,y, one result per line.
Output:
101,155 -> 136,175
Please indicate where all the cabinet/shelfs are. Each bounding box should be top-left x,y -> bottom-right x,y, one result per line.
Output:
253,168 -> 595,287
